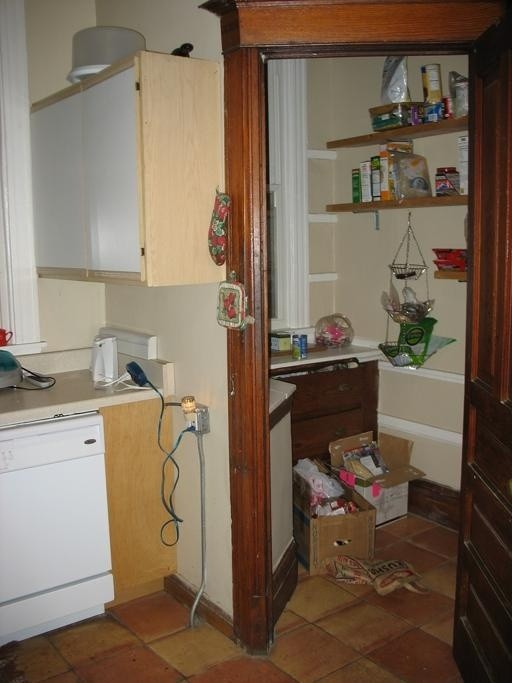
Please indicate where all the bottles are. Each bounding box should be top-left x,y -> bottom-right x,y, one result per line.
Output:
313,313 -> 354,347
292,334 -> 300,360
300,334 -> 307,359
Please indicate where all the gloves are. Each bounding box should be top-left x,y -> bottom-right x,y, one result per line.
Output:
208,194 -> 231,265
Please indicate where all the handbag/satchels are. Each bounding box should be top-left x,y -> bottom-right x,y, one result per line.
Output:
324,555 -> 431,596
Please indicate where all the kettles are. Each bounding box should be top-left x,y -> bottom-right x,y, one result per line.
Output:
91,333 -> 120,382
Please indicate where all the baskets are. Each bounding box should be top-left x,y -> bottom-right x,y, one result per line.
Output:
389,264 -> 428,280
379,341 -> 436,366
386,307 -> 432,323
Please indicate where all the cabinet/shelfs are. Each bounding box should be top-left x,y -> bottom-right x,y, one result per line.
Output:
326,117 -> 469,212
30,48 -> 225,287
100,393 -> 178,609
270,359 -> 378,468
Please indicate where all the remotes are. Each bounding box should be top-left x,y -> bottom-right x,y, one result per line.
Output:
24,370 -> 52,388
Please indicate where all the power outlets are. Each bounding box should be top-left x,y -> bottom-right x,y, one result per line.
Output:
183,404 -> 210,435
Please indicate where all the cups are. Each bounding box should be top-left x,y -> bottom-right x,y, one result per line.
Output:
0,328 -> 13,347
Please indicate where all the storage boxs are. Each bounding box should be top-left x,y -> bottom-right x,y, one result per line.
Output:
292,430 -> 426,576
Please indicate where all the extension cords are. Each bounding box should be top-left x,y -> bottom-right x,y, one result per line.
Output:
126,361 -> 147,387
